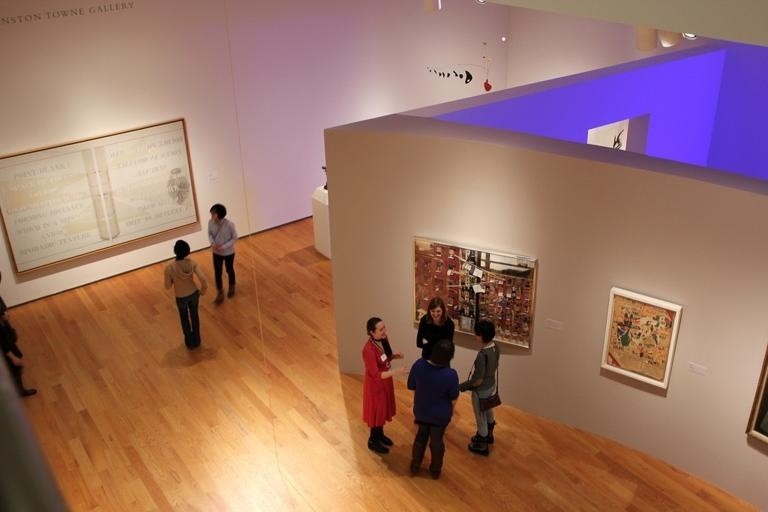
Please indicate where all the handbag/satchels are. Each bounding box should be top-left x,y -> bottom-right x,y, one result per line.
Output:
479,392 -> 501,412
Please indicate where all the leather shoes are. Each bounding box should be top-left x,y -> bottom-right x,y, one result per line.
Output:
368,435 -> 393,453
20,389 -> 35,398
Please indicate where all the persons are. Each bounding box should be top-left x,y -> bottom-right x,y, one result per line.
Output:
456,317 -> 501,458
359,316 -> 405,456
162,239 -> 208,350
207,203 -> 239,305
406,338 -> 460,481
413,297 -> 456,361
1,305 -> 38,398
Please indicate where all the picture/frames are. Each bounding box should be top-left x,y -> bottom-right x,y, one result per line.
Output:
413,237 -> 538,350
0,118 -> 201,274
599,286 -> 682,390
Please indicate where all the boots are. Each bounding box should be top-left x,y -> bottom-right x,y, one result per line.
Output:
471,421 -> 496,443
468,430 -> 490,456
215,289 -> 224,305
228,284 -> 235,298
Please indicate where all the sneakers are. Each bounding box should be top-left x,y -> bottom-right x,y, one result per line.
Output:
409,464 -> 418,473
429,464 -> 441,479
